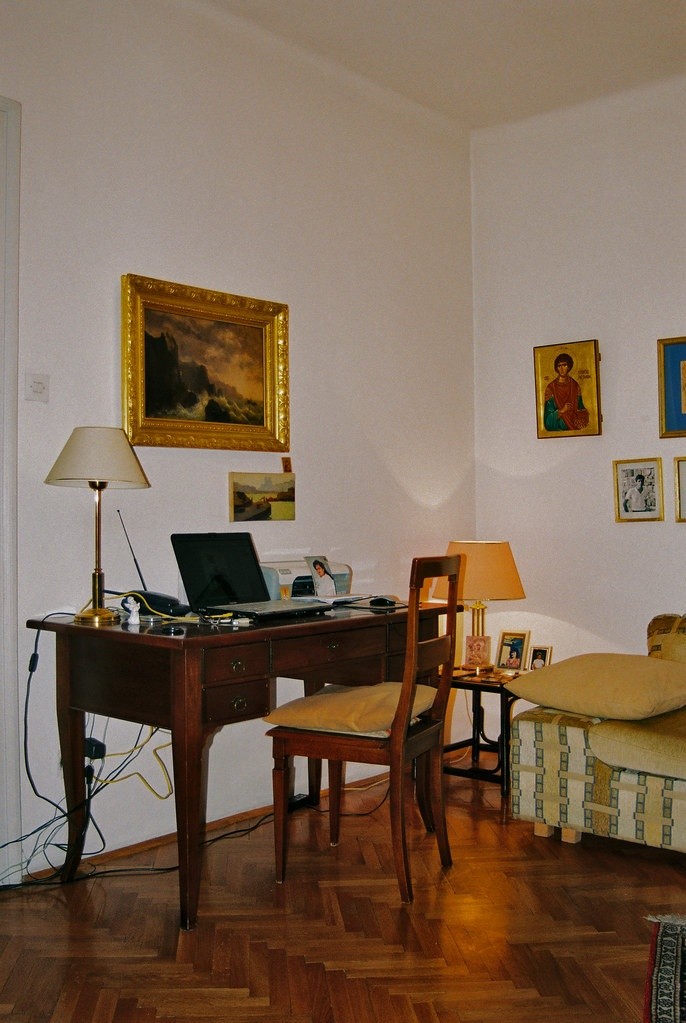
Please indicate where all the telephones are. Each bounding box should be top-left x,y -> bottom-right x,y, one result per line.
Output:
120,590 -> 195,621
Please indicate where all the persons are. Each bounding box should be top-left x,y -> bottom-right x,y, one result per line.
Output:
623,473 -> 649,512
312,560 -> 337,597
533,653 -> 545,670
126,596 -> 141,625
505,649 -> 520,668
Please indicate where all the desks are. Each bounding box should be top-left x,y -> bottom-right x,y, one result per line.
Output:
24,595 -> 461,932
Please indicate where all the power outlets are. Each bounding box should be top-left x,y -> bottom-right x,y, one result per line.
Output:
85,757 -> 102,783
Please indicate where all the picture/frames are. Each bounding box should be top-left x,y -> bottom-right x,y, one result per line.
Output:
118,273 -> 291,454
533,338 -> 603,440
673,457 -> 686,523
612,457 -> 666,524
493,629 -> 531,673
528,646 -> 553,672
656,335 -> 686,439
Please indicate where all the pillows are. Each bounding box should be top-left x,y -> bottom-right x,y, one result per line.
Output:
263,681 -> 437,732
502,650 -> 686,722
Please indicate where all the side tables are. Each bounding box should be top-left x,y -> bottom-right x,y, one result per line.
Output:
439,665 -> 523,796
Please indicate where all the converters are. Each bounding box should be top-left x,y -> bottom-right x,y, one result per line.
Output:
291,793 -> 309,813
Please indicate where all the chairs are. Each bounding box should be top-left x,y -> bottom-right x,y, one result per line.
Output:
264,553 -> 462,904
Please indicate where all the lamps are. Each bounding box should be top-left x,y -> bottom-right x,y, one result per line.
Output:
44,426 -> 150,625
431,539 -> 527,673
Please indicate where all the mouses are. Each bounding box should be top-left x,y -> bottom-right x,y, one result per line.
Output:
368,596 -> 395,606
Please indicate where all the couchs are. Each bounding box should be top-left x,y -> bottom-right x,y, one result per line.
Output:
511,612 -> 686,854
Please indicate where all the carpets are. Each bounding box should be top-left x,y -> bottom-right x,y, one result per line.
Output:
640,912 -> 686,1023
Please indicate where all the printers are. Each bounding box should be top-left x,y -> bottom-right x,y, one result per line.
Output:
259,560 -> 376,604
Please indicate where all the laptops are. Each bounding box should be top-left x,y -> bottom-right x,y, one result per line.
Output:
169,532 -> 337,621
